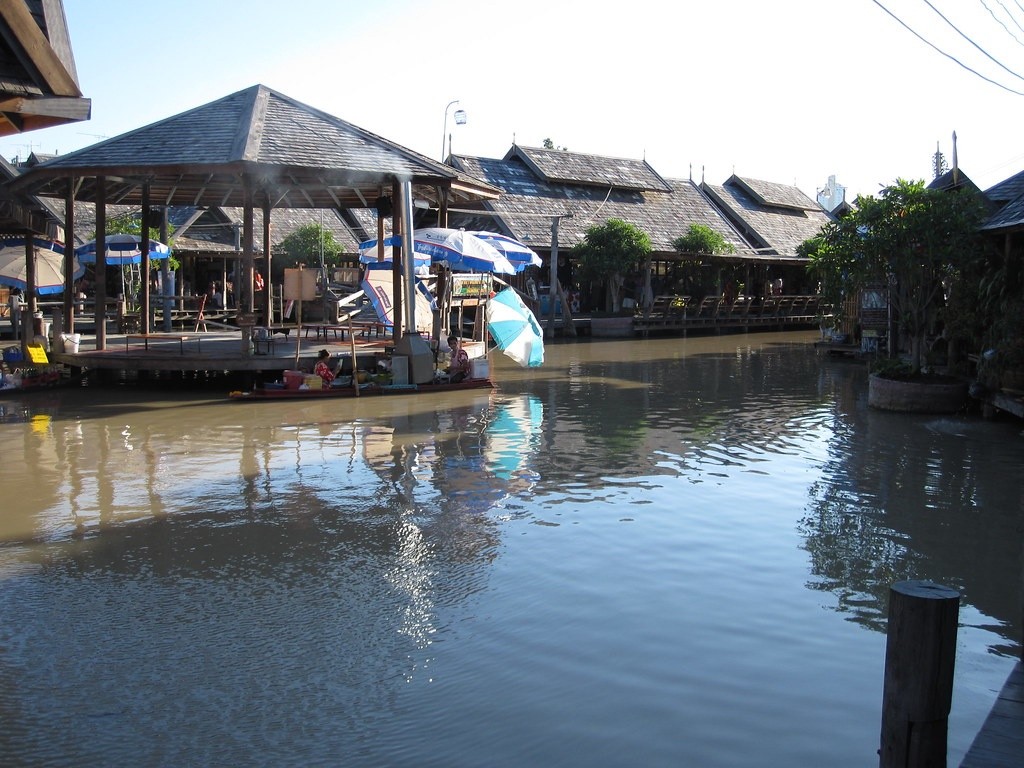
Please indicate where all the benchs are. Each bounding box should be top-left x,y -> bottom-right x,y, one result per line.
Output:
635,295 -> 842,319
233,323 -> 430,362
125,335 -> 201,356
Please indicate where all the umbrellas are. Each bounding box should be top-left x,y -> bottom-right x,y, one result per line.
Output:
0,227 -> 85,309
359,224 -> 542,338
354,262 -> 436,338
466,286 -> 546,371
76,232 -> 172,315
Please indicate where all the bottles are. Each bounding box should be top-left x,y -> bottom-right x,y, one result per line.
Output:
356,369 -> 368,385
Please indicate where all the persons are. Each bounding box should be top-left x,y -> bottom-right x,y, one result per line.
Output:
313,349 -> 342,388
375,345 -> 394,376
442,335 -> 470,385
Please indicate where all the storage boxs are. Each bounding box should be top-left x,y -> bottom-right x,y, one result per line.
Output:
303,376 -> 322,389
470,359 -> 489,378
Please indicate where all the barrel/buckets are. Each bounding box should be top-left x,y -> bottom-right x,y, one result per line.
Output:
263,382 -> 284,389
2,350 -> 23,362
283,369 -> 303,390
61,333 -> 81,354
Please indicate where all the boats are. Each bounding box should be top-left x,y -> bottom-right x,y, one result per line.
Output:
230,378 -> 494,403
